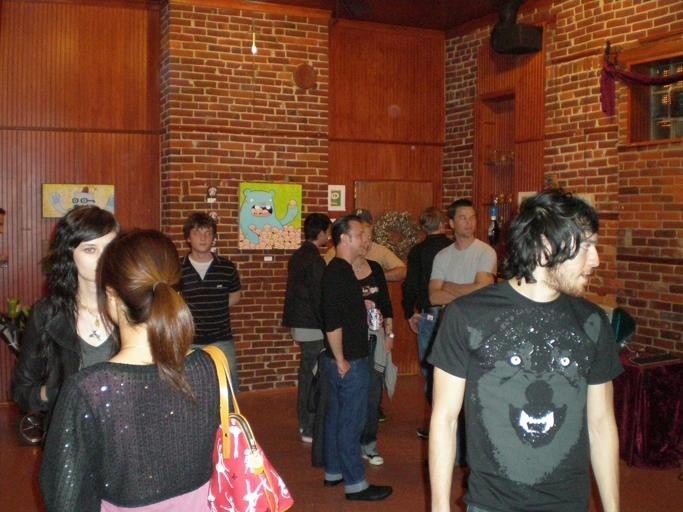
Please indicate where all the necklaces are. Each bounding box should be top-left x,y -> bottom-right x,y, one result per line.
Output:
80,300 -> 105,328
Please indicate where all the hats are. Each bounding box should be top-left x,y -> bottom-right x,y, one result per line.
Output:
351,208 -> 373,225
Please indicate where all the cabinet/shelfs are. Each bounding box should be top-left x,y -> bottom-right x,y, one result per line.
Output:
481,157 -> 514,206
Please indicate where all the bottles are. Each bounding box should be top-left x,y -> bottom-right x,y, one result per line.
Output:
487,203 -> 500,246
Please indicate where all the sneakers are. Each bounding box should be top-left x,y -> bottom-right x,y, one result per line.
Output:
298,427 -> 313,445
323,477 -> 344,488
361,450 -> 384,466
344,483 -> 393,502
378,409 -> 388,423
416,424 -> 429,440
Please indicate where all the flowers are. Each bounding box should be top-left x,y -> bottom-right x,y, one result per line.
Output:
372,210 -> 421,260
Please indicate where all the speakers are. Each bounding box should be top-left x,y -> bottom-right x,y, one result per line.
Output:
493,24 -> 541,53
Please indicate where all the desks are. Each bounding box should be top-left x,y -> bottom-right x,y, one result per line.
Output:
611,343 -> 683,473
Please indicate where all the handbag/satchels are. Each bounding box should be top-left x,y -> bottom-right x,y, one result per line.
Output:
202,344 -> 294,512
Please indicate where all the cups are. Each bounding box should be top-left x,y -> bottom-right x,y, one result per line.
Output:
366,308 -> 381,331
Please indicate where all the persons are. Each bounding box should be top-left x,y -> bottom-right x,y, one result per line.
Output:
0,207 -> 9,262
402,198 -> 498,464
11,204 -> 121,416
285,211 -> 407,501
423,187 -> 627,512
38,226 -> 241,512
177,211 -> 241,412
